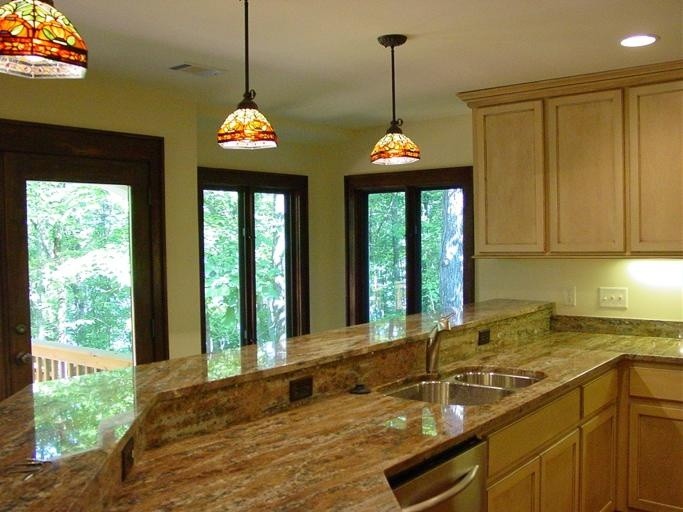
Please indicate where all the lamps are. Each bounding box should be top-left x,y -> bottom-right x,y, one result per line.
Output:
217,0 -> 281,149
0,0 -> 89,80
370,34 -> 420,166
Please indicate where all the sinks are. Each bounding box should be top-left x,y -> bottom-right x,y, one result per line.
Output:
380,377 -> 515,408
445,362 -> 548,393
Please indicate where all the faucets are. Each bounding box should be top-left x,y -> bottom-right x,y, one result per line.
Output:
422,314 -> 451,375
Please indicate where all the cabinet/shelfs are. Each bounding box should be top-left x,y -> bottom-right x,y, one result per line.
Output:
480,361 -> 683,512
456,58 -> 683,258
